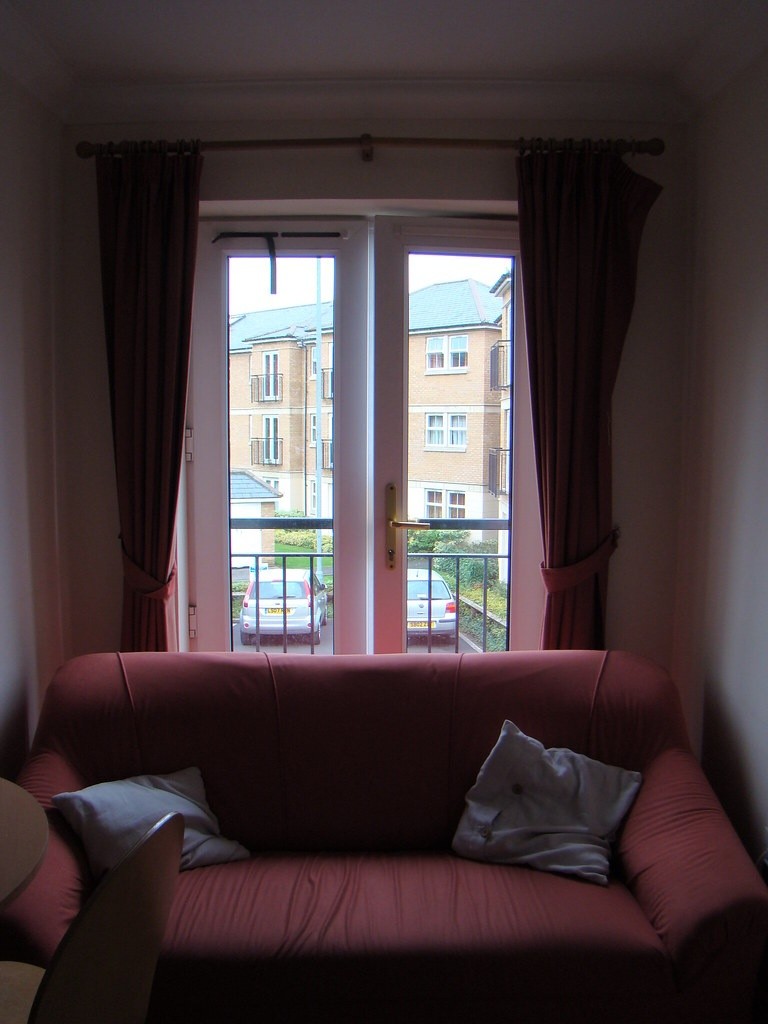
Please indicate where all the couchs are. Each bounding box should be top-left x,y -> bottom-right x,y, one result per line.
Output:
0,649 -> 768,1024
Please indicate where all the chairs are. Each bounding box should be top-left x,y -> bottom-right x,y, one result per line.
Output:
0,811 -> 185,1024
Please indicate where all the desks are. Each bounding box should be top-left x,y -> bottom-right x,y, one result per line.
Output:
0,778 -> 48,912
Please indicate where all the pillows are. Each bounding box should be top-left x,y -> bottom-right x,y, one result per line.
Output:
50,766 -> 251,881
449,720 -> 643,886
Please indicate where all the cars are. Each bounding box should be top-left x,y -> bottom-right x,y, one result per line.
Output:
407,569 -> 459,643
240,568 -> 328,645
